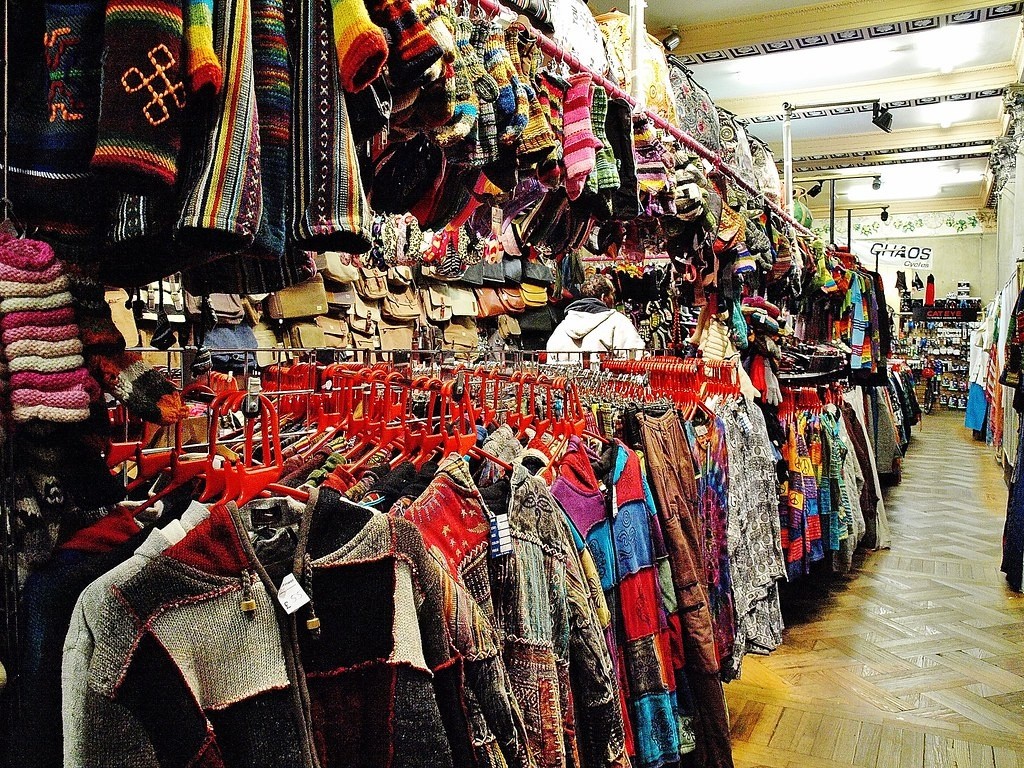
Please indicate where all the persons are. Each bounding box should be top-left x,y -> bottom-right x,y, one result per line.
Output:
542,273 -> 650,361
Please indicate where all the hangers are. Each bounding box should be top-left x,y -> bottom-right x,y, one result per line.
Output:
1,0 -> 922,766
